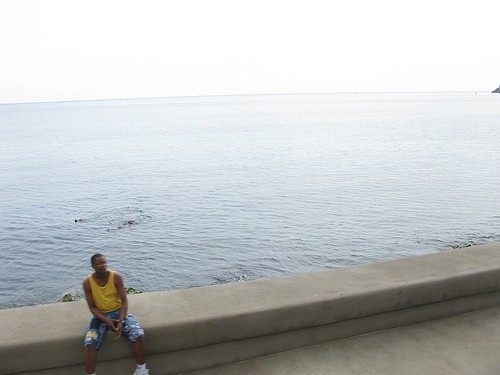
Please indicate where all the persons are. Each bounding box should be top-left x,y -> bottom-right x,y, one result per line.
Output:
81,253 -> 149,375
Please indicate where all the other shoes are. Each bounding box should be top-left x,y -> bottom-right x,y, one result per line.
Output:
133,368 -> 150,375
